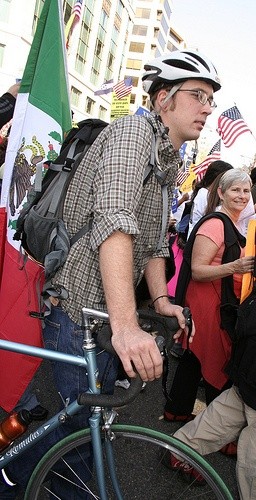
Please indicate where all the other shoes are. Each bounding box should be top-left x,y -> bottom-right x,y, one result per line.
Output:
163,408 -> 196,423
157,436 -> 207,488
220,436 -> 240,458
26,405 -> 50,421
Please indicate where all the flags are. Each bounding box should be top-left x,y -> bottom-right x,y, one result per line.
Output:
0,0 -> 75,413
177,159 -> 191,185
190,138 -> 223,186
112,75 -> 133,100
94,79 -> 114,96
71,0 -> 83,19
215,106 -> 253,148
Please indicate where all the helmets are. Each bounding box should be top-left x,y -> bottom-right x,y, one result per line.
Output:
140,50 -> 221,93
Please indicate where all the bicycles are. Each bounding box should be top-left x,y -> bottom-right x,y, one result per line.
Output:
0,305 -> 233,500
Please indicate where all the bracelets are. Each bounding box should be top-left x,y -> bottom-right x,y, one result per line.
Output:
148,295 -> 169,309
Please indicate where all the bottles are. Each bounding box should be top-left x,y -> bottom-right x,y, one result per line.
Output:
0,404 -> 49,451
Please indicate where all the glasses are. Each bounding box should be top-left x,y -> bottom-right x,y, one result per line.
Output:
166,89 -> 215,108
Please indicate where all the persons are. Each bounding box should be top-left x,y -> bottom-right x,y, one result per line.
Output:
0,79 -> 22,165
0,49 -> 222,500
162,160 -> 256,500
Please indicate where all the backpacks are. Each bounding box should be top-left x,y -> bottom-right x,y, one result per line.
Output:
224,285 -> 256,410
11,118 -> 159,275
